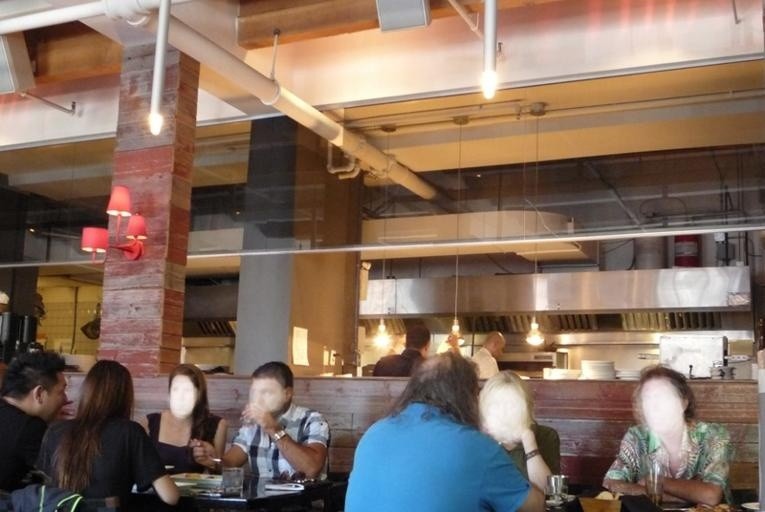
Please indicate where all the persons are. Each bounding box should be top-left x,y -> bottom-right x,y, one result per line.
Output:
188,362 -> 332,511
344,351 -> 546,512
34,359 -> 182,507
602,363 -> 733,505
0,352 -> 76,493
472,332 -> 507,380
137,364 -> 228,474
372,326 -> 433,377
478,370 -> 560,495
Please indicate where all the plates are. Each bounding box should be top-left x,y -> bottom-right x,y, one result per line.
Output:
542,358 -> 643,382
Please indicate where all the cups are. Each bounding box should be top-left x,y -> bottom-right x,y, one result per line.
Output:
220,466 -> 245,498
647,454 -> 666,506
542,472 -> 572,505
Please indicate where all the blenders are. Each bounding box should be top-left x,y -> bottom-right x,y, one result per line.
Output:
0,311 -> 36,363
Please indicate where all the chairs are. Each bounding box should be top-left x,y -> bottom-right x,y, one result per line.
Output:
0,492 -> 121,512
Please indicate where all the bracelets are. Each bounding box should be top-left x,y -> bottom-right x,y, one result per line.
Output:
524,448 -> 542,462
272,429 -> 286,442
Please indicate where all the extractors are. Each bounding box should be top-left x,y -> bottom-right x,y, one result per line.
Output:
183,284 -> 238,341
354,264 -> 757,347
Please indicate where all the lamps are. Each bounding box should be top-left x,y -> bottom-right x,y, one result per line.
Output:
525,116 -> 544,346
145,0 -> 172,135
80,185 -> 148,264
481,1 -> 500,100
372,132 -> 392,351
446,125 -> 465,346
